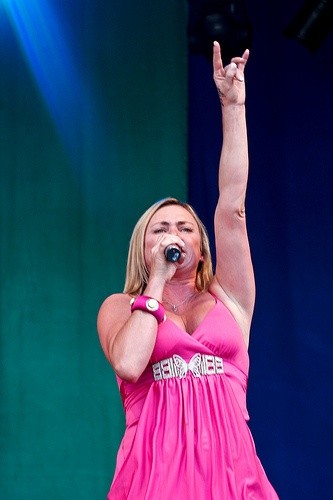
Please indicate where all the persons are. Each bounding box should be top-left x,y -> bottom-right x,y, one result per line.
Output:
128,295 -> 167,325
94,39 -> 280,500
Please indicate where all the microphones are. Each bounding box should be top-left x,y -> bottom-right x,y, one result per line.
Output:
164,243 -> 181,262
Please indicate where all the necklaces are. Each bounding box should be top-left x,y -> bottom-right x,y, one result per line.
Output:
161,291 -> 196,312
